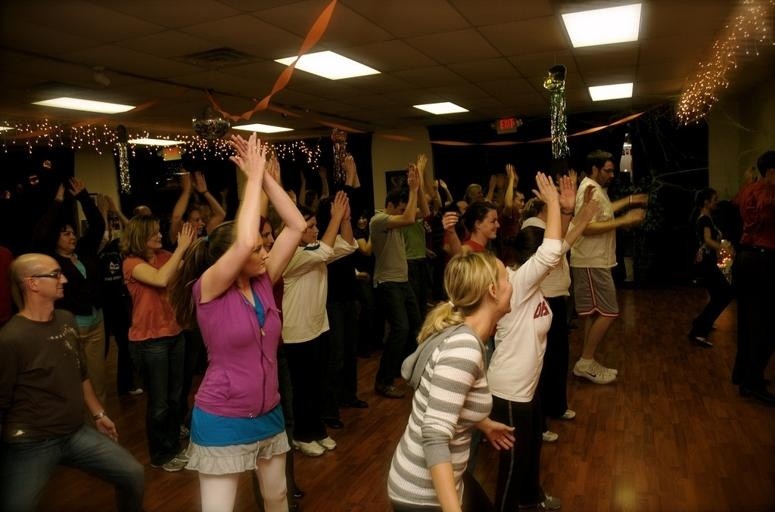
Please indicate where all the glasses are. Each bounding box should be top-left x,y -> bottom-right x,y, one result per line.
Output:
31,270 -> 62,279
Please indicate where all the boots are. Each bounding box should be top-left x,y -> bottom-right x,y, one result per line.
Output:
253,473 -> 303,512
285,461 -> 306,500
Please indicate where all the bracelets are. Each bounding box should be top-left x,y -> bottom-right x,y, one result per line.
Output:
628,196 -> 633,208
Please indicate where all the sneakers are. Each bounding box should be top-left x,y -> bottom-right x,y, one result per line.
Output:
542,430 -> 559,443
291,435 -> 326,457
174,448 -> 193,462
374,381 -> 405,398
149,456 -> 185,472
561,409 -> 576,419
573,360 -> 618,384
518,495 -> 562,510
318,436 -> 337,450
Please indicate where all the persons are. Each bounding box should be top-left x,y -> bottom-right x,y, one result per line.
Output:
688,187 -> 733,348
569,149 -> 652,386
731,151 -> 775,407
0,132 -> 600,508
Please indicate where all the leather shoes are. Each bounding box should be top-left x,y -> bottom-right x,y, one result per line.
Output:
688,330 -> 713,348
129,388 -> 143,395
737,384 -> 774,406
339,398 -> 368,408
323,419 -> 344,429
